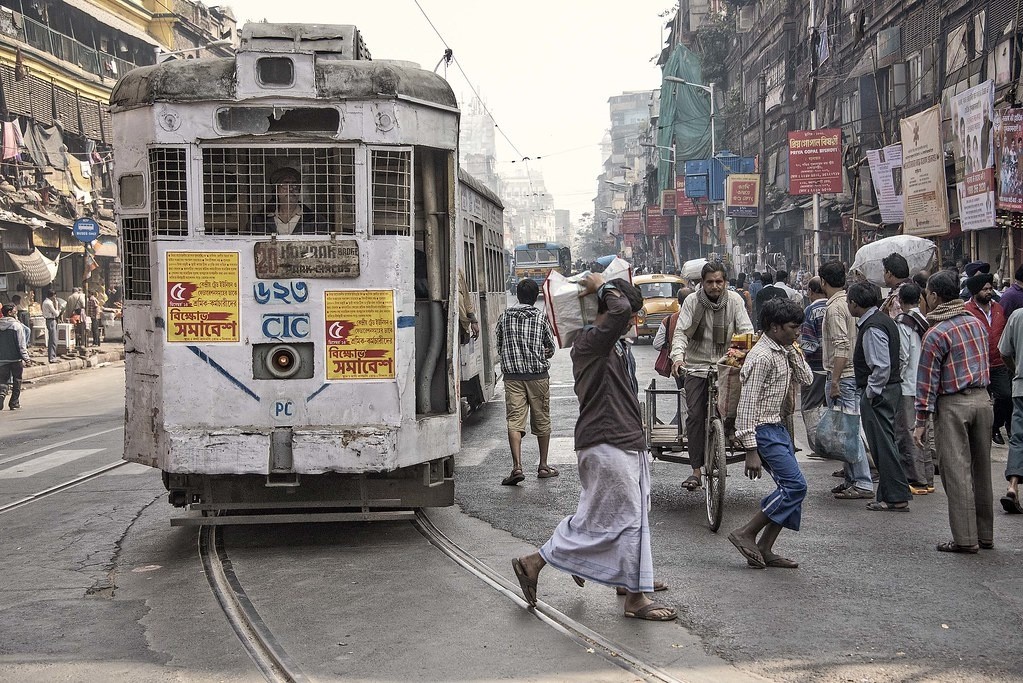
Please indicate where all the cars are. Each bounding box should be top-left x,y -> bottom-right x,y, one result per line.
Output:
630,274 -> 692,344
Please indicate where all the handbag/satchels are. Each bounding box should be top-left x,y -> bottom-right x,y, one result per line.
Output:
654,342 -> 674,378
717,347 -> 796,419
541,257 -> 640,349
803,395 -> 870,464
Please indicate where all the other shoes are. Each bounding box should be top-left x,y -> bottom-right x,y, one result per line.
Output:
0,395 -> 4,410
49,357 -> 62,363
10,404 -> 23,410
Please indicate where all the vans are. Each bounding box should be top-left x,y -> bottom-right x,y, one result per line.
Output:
512,242 -> 572,297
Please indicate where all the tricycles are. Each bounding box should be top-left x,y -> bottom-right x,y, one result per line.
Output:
642,365 -> 802,531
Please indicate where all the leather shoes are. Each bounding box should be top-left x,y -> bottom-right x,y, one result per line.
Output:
991,429 -> 1005,445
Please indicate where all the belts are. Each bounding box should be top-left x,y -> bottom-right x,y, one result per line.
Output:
46,318 -> 55,320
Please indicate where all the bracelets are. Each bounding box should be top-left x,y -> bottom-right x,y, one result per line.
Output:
746,447 -> 758,452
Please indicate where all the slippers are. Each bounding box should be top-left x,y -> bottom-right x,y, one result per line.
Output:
616,580 -> 669,596
1000,496 -> 1023,514
937,535 -> 994,554
831,463 -> 939,513
748,555 -> 799,569
572,572 -> 584,587
681,476 -> 703,488
728,533 -> 766,568
512,557 -> 538,607
624,601 -> 678,621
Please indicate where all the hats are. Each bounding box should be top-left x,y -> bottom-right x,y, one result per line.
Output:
963,260 -> 993,295
270,167 -> 301,189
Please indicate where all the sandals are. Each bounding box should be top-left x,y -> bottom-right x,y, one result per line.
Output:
501,468 -> 526,485
537,466 -> 559,478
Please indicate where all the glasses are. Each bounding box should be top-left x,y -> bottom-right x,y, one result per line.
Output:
278,182 -> 300,191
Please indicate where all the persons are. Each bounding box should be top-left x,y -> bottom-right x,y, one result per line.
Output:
1,305 -> 31,410
846,285 -> 914,512
457,265 -> 480,345
803,276 -> 830,460
819,260 -> 874,499
953,99 -> 1022,209
574,258 -> 1022,298
877,253 -> 925,318
572,340 -> 669,595
893,284 -> 936,495
652,288 -> 694,437
66,285 -> 102,345
963,274 -> 1012,445
245,168 -> 334,235
495,279 -> 559,486
7,295 -> 31,347
670,262 -> 754,489
727,296 -> 815,568
909,270 -> 995,554
510,271 -> 678,621
997,308 -> 1023,514
15,283 -> 34,328
42,288 -> 61,362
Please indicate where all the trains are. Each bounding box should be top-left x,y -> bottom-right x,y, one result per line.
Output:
105,23 -> 513,527
